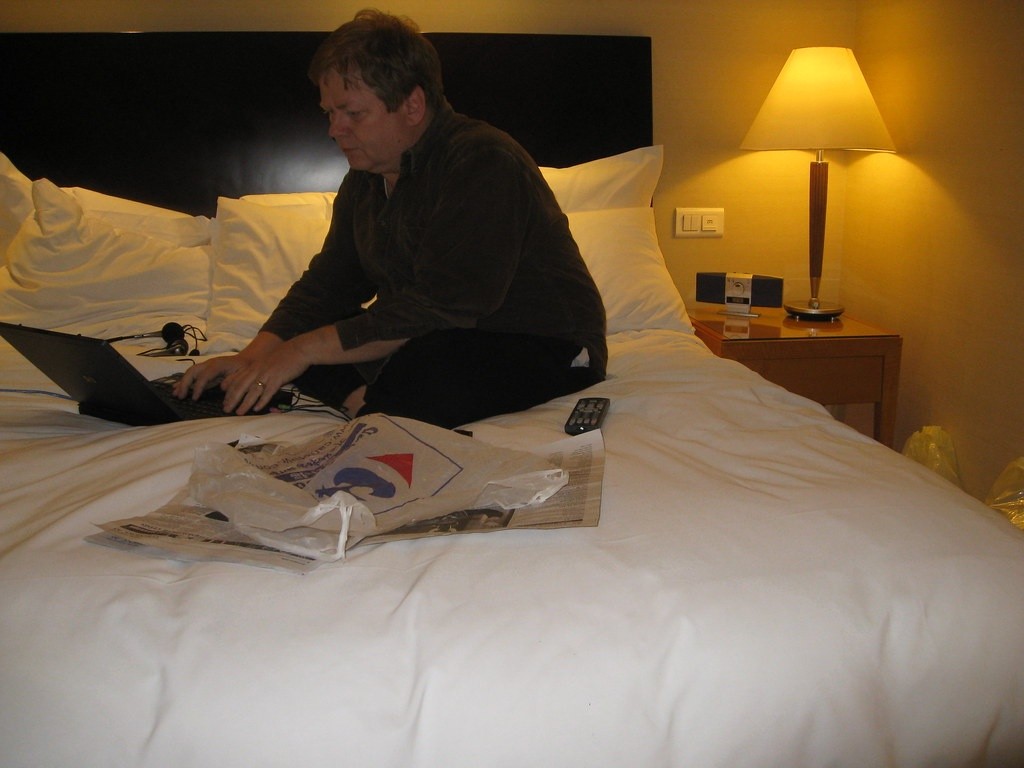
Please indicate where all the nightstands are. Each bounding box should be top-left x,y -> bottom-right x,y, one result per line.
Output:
685,300 -> 903,449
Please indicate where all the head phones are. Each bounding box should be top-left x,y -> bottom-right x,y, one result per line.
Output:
106,323 -> 189,357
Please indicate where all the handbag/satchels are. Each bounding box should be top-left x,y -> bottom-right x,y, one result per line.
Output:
184,409 -> 571,561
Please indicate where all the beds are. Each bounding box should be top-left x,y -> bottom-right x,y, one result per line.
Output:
0,31 -> 1024,768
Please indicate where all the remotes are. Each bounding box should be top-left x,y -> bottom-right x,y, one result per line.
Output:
564,397 -> 609,436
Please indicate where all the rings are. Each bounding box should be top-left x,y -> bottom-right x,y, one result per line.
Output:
254,379 -> 266,387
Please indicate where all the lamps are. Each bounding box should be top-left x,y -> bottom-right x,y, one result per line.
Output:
738,47 -> 898,317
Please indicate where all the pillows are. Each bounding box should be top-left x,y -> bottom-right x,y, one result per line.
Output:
564,208 -> 696,336
0,152 -> 213,269
537,145 -> 664,213
203,196 -> 378,356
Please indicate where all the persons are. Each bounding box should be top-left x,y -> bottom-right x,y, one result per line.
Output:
172,6 -> 607,430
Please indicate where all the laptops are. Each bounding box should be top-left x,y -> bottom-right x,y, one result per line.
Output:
0,322 -> 294,427
